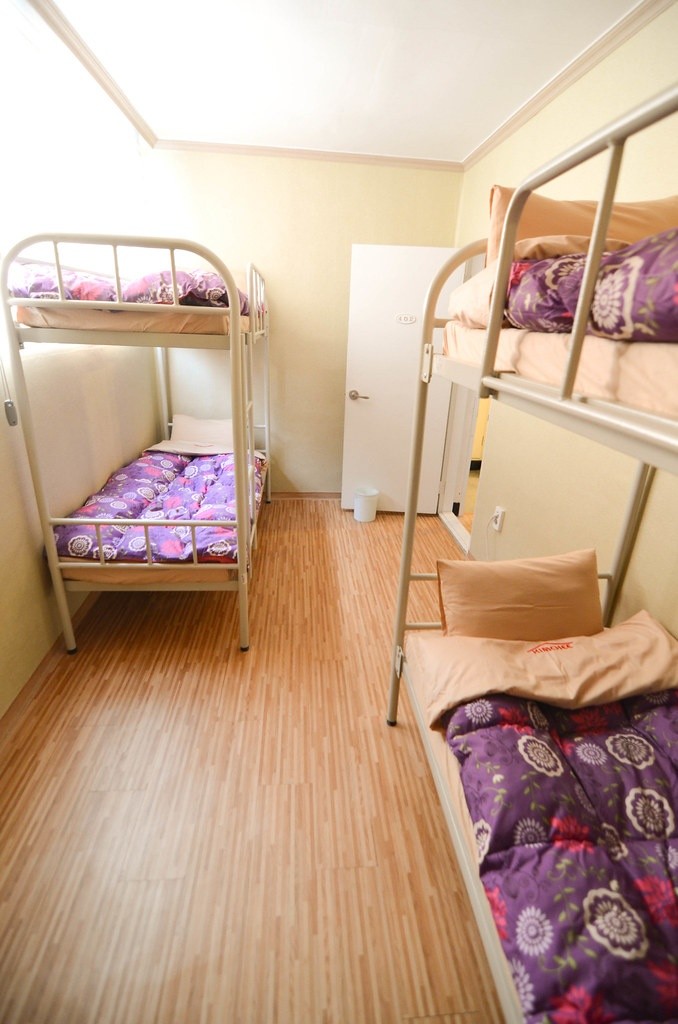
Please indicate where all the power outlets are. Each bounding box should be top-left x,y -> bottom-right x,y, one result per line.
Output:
493,506 -> 505,533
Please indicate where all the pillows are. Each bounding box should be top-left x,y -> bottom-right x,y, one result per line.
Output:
487,183 -> 678,267
436,546 -> 604,641
171,413 -> 250,448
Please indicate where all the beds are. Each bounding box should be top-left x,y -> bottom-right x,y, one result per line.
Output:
387,85 -> 678,1024
0,230 -> 274,654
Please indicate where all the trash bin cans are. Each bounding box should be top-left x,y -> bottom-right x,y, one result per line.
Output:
354,487 -> 379,522
